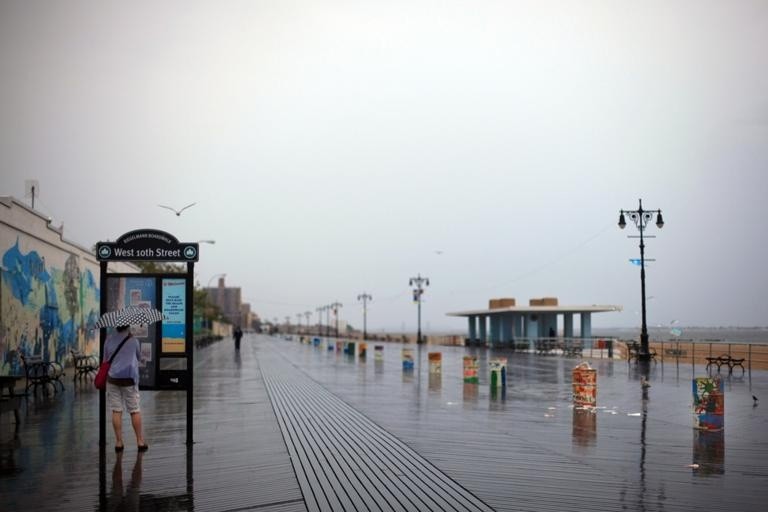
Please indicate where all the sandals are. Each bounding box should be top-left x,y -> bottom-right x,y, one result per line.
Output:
114,445 -> 124,452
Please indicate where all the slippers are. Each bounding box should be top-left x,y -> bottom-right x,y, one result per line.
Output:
138,444 -> 148,450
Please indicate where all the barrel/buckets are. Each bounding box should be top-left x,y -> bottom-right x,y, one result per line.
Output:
691,377 -> 724,434
299,336 -> 367,358
375,345 -> 383,358
572,361 -> 598,410
402,348 -> 416,368
695,431 -> 725,477
429,353 -> 442,374
428,372 -> 441,390
490,385 -> 507,402
463,381 -> 478,401
462,356 -> 480,383
572,406 -> 598,444
488,358 -> 507,387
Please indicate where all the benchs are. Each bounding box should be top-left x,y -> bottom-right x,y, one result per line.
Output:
628,346 -> 657,364
21,356 -> 66,393
704,354 -> 745,375
71,351 -> 98,382
0,375 -> 31,433
509,340 -> 584,358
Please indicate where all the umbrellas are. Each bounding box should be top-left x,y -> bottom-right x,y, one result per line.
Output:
94,306 -> 170,326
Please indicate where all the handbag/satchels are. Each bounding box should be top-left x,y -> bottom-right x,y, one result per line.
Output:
94,361 -> 110,389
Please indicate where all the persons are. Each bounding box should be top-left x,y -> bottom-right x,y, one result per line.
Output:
103,324 -> 149,453
234,326 -> 243,349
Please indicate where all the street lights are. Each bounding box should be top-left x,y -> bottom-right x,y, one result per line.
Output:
284,298 -> 344,337
617,198 -> 665,361
408,272 -> 430,344
357,290 -> 373,341
204,272 -> 228,329
